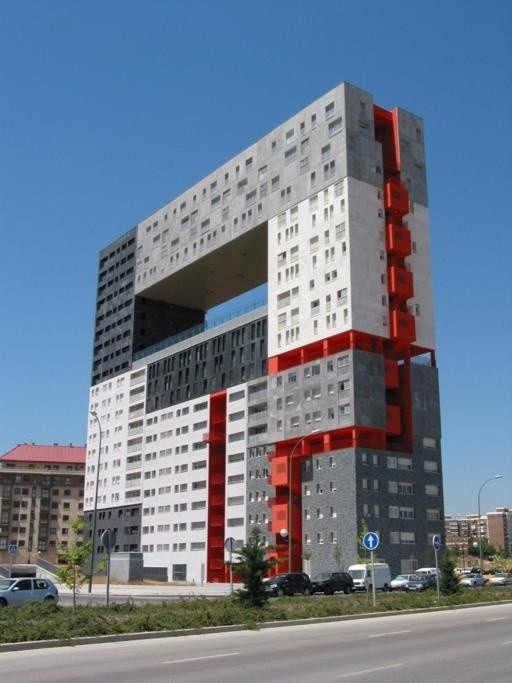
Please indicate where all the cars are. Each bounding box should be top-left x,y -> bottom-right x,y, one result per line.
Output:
0,577 -> 59,608
262,562 -> 441,597
456,568 -> 512,587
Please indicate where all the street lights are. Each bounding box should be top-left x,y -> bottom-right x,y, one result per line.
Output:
289,428 -> 321,573
478,475 -> 504,571
88,410 -> 102,593
